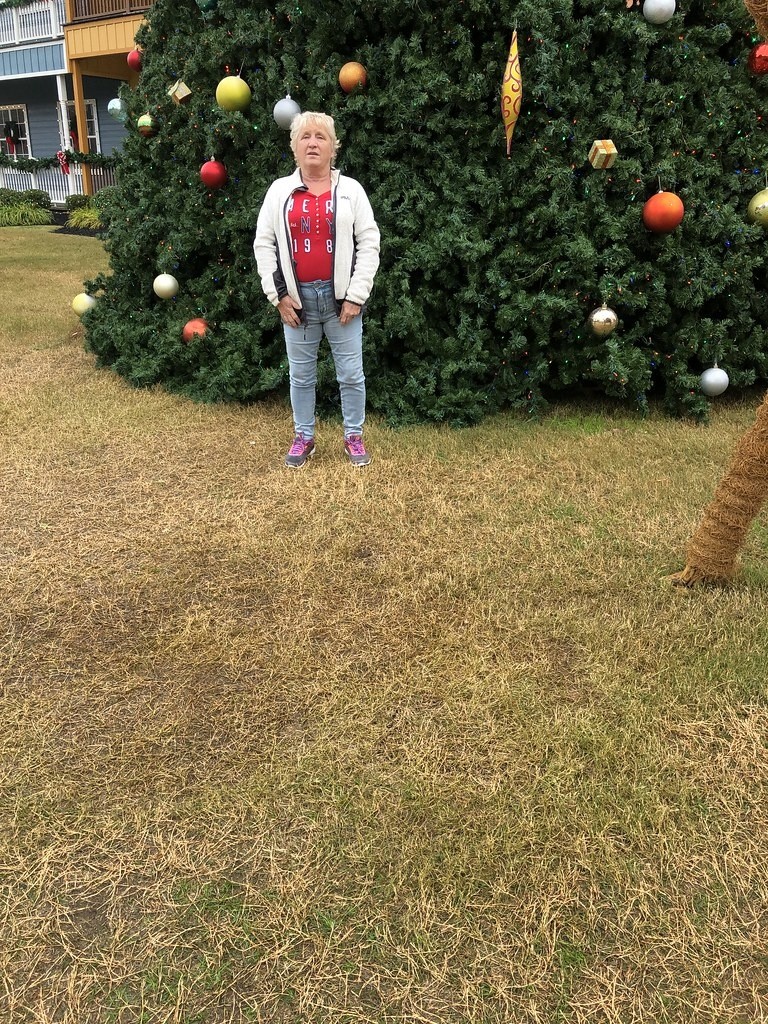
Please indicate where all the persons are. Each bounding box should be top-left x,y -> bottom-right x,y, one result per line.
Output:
253,110 -> 380,468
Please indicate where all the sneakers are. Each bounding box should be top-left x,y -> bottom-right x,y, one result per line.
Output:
343,434 -> 372,466
284,432 -> 316,469
